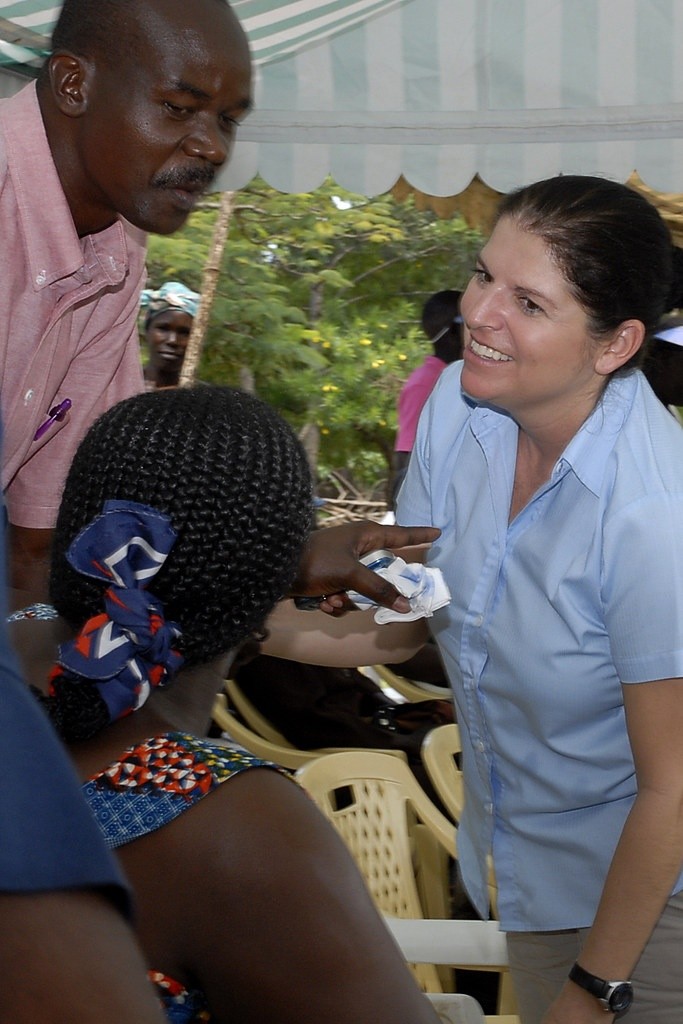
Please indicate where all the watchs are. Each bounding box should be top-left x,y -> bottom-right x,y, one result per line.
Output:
568,963 -> 634,1013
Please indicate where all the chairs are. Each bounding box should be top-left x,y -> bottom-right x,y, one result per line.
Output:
211,663 -> 522,1024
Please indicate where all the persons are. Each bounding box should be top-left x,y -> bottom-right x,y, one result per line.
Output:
0,0 -> 683,1024
